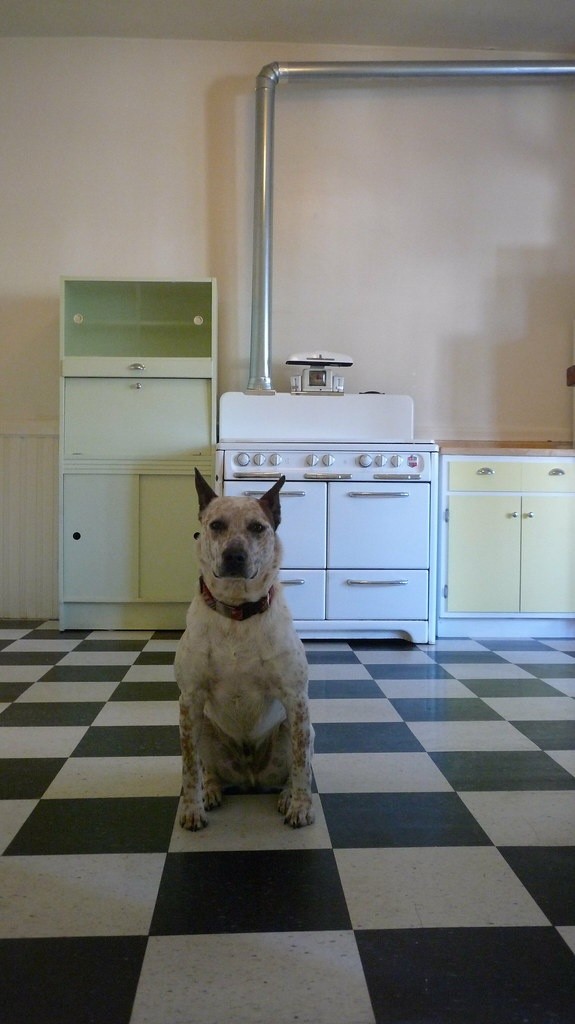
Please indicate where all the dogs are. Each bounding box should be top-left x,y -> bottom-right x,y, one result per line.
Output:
172,466 -> 315,831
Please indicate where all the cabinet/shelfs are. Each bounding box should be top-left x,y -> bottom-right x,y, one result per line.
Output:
436,454 -> 575,638
56,275 -> 218,632
